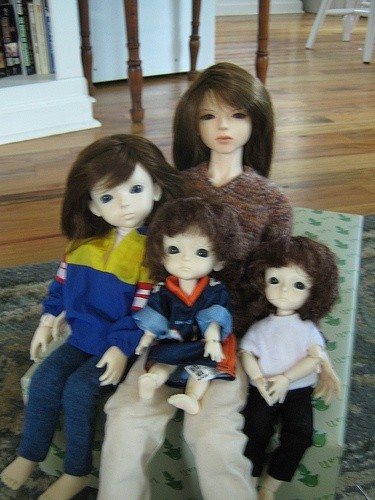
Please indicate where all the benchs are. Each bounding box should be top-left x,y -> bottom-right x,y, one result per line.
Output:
21,207 -> 363,500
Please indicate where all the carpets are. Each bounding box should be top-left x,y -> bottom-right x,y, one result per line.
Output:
0,213 -> 375,499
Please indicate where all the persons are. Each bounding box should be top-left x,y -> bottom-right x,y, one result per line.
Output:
50,63 -> 342,500
239,236 -> 338,500
134,197 -> 244,414
0,135 -> 173,500
